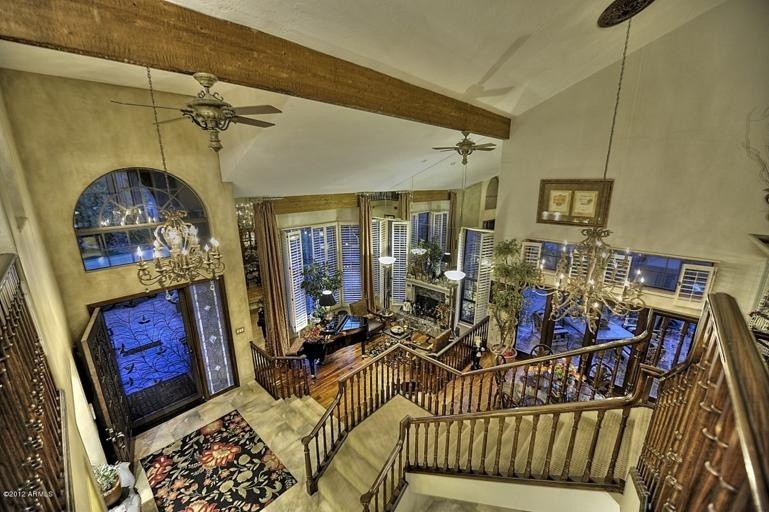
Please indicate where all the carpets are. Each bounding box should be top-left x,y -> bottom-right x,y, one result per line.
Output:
431,352 -> 618,414
139,409 -> 297,512
366,335 -> 417,370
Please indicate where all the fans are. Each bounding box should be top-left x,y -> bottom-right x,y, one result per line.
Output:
112,74 -> 282,130
430,129 -> 497,154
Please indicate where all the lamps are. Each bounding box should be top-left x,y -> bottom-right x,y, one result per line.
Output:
131,66 -> 228,304
532,1 -> 655,336
318,290 -> 335,320
460,154 -> 469,164
444,270 -> 466,340
207,129 -> 225,152
377,256 -> 397,324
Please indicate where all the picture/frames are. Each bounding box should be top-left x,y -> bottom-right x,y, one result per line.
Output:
535,179 -> 616,226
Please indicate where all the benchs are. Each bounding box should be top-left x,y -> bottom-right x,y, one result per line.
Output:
615,326 -> 664,366
533,309 -> 574,350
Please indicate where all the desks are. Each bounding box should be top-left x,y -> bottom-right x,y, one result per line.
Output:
560,316 -> 635,354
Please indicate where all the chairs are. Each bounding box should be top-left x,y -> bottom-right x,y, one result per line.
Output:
349,298 -> 385,345
403,328 -> 452,353
491,343 -> 615,410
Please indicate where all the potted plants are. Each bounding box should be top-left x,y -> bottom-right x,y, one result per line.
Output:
490,239 -> 542,367
90,465 -> 126,508
